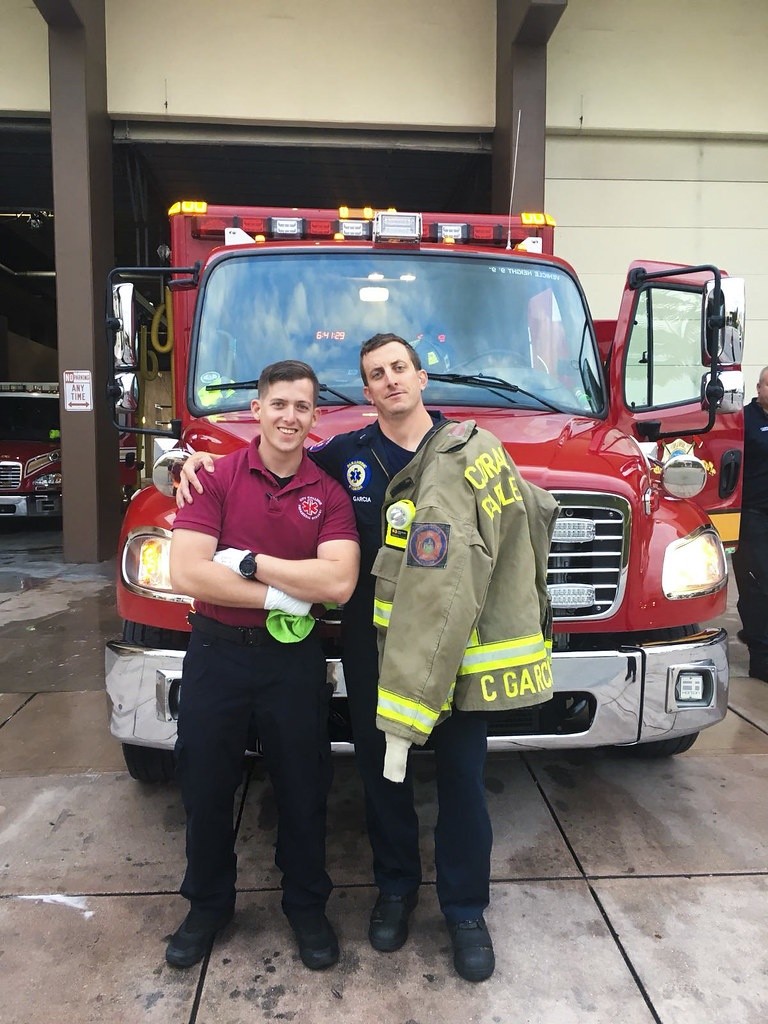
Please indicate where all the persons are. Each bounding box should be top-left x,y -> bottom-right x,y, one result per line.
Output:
166,358 -> 363,971
176,334 -> 495,983
731,367 -> 768,687
367,303 -> 453,377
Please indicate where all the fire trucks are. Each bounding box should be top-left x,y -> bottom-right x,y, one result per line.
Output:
104,201 -> 748,785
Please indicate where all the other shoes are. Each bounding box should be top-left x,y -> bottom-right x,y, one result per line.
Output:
737,629 -> 747,643
749,656 -> 767,682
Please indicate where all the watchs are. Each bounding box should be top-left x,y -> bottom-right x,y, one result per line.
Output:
240,551 -> 258,581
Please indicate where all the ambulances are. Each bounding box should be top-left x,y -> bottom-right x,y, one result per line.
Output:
0,380 -> 145,519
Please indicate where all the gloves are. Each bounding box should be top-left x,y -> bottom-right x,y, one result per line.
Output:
264,586 -> 313,617
211,548 -> 251,578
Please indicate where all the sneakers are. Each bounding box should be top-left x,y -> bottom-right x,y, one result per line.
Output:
166,905 -> 234,968
445,910 -> 497,982
367,876 -> 422,952
286,910 -> 339,969
